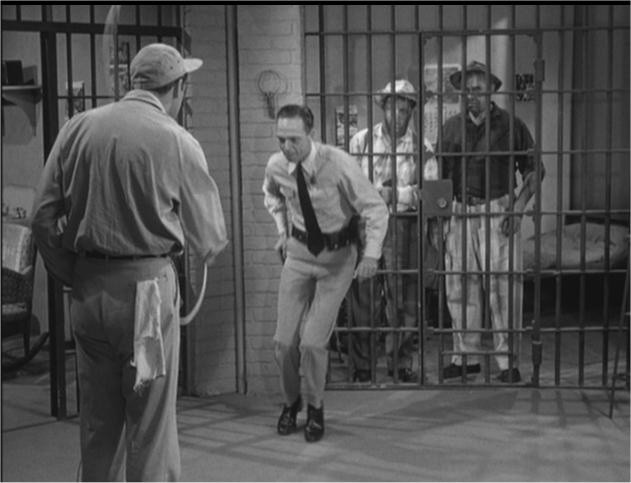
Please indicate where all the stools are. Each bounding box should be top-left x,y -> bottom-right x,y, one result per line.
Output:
84,250 -> 168,260
451,192 -> 509,205
291,227 -> 356,248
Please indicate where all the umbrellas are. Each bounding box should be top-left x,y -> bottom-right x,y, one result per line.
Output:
278,396 -> 303,433
388,368 -> 416,382
502,368 -> 519,383
353,374 -> 372,382
305,401 -> 324,443
444,362 -> 480,377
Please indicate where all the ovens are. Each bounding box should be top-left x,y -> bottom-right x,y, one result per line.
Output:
521,217 -> 631,278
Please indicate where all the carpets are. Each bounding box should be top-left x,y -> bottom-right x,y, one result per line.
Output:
295,163 -> 324,258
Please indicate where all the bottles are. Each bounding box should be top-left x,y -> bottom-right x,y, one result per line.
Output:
450,61 -> 502,92
374,80 -> 427,110
128,43 -> 204,90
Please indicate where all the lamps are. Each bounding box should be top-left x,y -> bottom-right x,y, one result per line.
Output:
1,219 -> 36,368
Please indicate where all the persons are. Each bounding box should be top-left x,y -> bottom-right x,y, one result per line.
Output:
433,59 -> 549,384
29,38 -> 231,482
263,102 -> 397,440
349,77 -> 440,384
